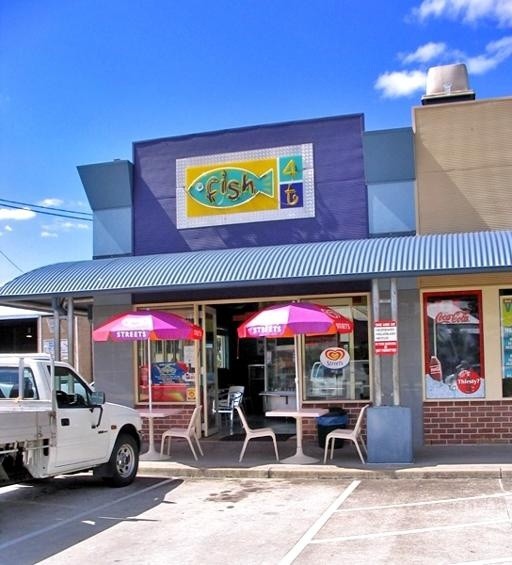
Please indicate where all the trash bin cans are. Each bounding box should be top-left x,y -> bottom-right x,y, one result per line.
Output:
318,407 -> 347,449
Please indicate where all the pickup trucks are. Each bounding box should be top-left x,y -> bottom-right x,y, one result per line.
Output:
1,353 -> 144,488
308,360 -> 369,399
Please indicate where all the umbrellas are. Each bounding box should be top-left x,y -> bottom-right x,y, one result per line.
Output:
91,307 -> 205,449
235,300 -> 355,450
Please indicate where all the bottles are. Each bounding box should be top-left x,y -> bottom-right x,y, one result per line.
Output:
429,355 -> 442,382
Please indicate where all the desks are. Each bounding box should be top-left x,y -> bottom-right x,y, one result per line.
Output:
265,407 -> 331,464
135,408 -> 180,460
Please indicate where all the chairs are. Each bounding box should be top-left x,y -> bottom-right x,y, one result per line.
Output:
159,403 -> 205,462
212,383 -> 245,437
234,406 -> 283,465
322,404 -> 369,462
